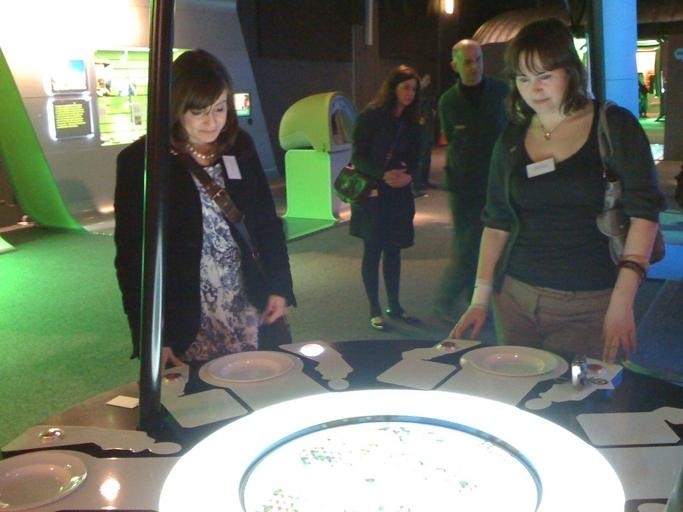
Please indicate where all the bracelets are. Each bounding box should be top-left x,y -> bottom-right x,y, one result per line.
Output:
616,253 -> 652,288
472,282 -> 495,293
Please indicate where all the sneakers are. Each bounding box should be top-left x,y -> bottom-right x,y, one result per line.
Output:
387,307 -> 419,323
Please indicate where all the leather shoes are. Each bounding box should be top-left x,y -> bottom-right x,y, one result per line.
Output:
370,314 -> 385,330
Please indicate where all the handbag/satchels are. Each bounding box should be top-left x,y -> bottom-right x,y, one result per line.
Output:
256,250 -> 292,348
597,100 -> 666,265
334,166 -> 378,206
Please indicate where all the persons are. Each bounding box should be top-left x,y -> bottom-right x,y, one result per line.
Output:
635,71 -> 648,119
107,47 -> 294,367
436,36 -> 511,313
673,165 -> 682,210
344,64 -> 426,335
444,16 -> 666,366
416,69 -> 433,188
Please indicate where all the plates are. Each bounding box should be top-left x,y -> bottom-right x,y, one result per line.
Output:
206,351 -> 295,382
0,452 -> 88,511
470,347 -> 558,377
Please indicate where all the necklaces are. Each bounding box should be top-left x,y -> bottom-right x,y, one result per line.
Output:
180,138 -> 220,161
533,112 -> 567,141
